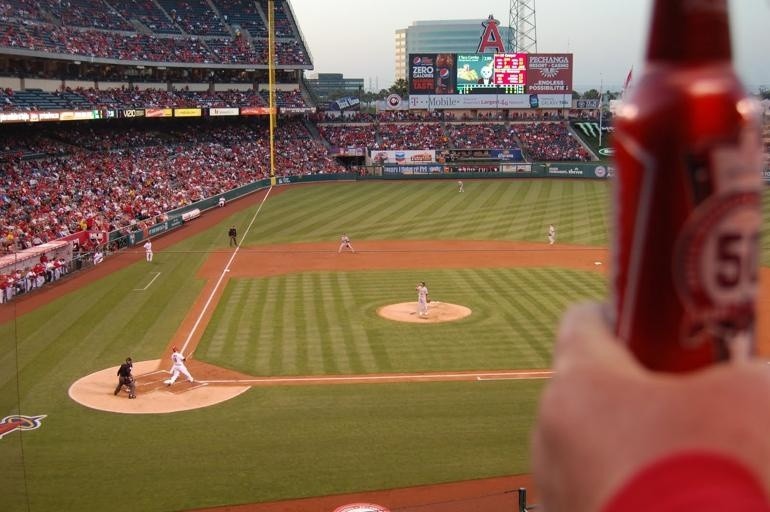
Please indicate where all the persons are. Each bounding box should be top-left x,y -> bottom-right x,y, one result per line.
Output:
316,110 -> 589,162
416,280 -> 431,315
114,357 -> 136,399
338,232 -> 355,253
1,2 -> 346,299
167,344 -> 193,387
533,303 -> 768,511
548,224 -> 556,244
457,180 -> 464,192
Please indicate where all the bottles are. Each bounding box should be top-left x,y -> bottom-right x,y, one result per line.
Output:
435,54 -> 455,94
607,1 -> 766,376
